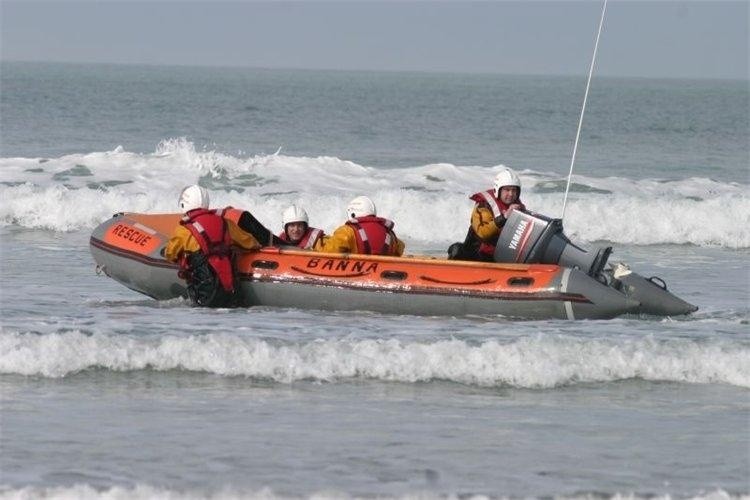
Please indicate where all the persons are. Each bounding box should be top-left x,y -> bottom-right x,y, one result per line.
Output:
452,168 -> 527,263
316,194 -> 405,257
160,184 -> 263,308
278,203 -> 329,252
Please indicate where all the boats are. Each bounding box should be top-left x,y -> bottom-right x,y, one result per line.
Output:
91,206 -> 698,319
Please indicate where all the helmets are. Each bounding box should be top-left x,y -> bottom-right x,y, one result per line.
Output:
179,186 -> 210,213
345,195 -> 377,219
492,170 -> 522,201
281,205 -> 309,228
